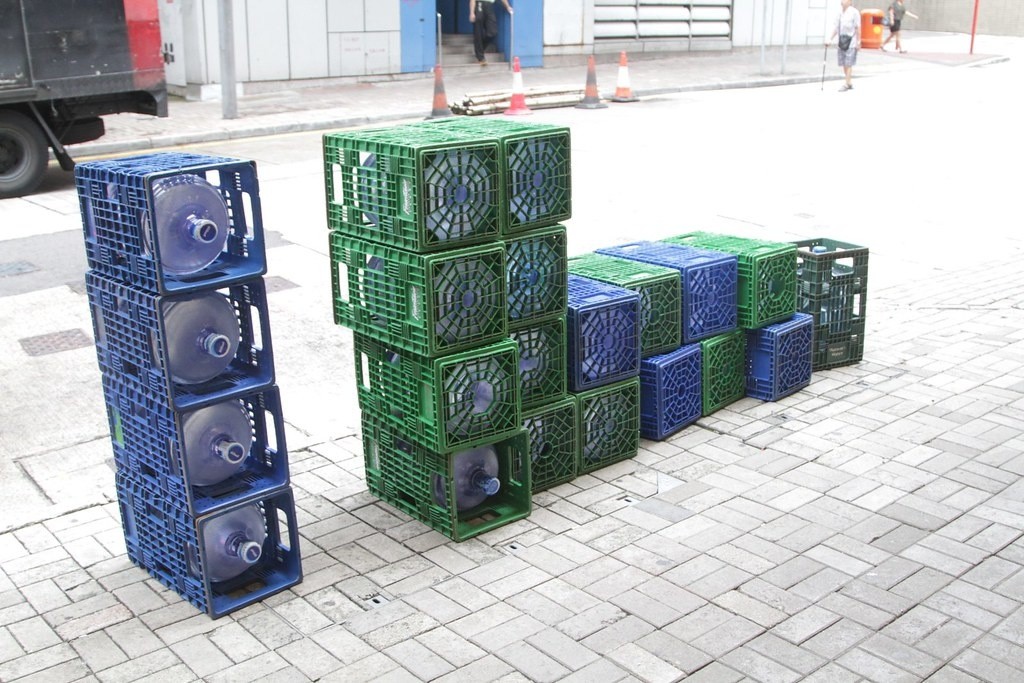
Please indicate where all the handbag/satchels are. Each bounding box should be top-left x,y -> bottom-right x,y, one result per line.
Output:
838,34 -> 852,50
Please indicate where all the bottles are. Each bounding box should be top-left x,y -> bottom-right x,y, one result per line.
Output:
111,402 -> 252,488
85,174 -> 227,275
123,501 -> 267,585
364,256 -> 504,341
358,152 -> 491,241
94,273 -> 240,385
797,246 -> 848,365
382,348 -> 506,430
375,441 -> 501,512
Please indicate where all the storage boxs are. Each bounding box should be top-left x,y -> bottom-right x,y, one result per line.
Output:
73,151 -> 268,295
402,115 -> 572,235
328,231 -> 509,358
361,410 -> 532,543
568,230 -> 814,477
788,238 -> 869,371
101,374 -> 291,518
508,314 -> 567,411
521,394 -> 578,495
322,124 -> 505,254
84,270 -> 275,412
115,471 -> 303,619
496,223 -> 569,329
353,330 -> 521,454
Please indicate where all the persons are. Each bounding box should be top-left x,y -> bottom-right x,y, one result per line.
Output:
824,0 -> 860,92
469,0 -> 513,64
880,0 -> 917,53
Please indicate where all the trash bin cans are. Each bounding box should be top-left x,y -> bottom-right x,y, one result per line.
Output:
860,7 -> 885,49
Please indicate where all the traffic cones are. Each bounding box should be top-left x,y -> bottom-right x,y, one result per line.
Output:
503,58 -> 533,114
422,64 -> 454,119
575,55 -> 608,109
611,52 -> 640,103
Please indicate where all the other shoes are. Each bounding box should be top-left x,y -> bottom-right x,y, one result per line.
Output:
899,49 -> 906,53
479,58 -> 488,66
878,46 -> 887,52
838,84 -> 853,92
